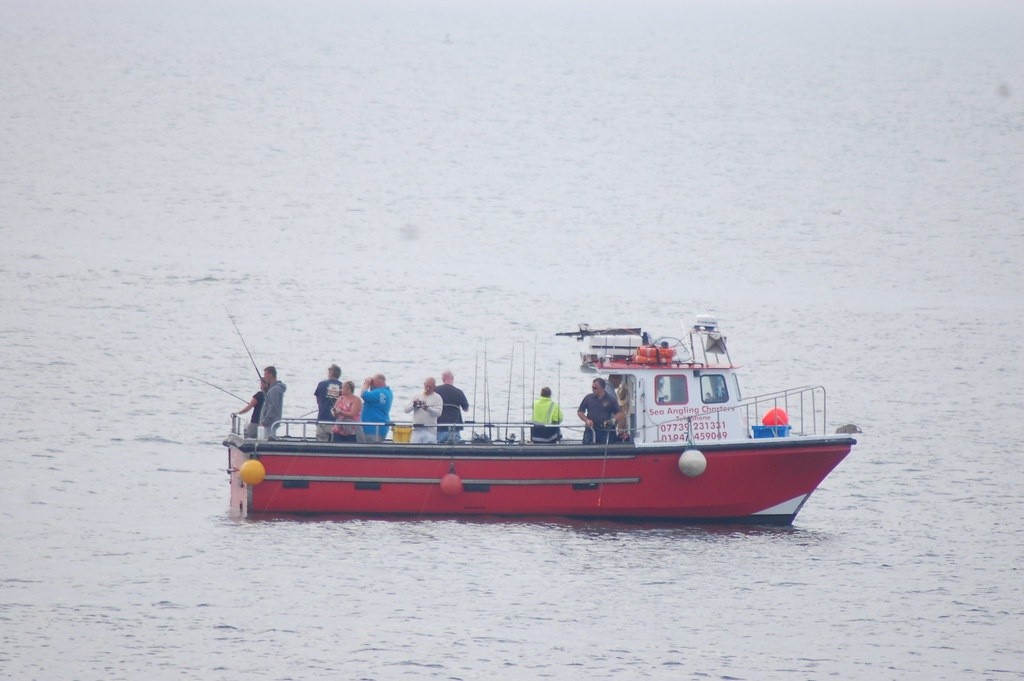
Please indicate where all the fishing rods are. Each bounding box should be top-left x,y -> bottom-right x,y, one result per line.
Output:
468,333 -> 563,442
595,422 -> 615,529
169,372 -> 249,405
222,306 -> 264,381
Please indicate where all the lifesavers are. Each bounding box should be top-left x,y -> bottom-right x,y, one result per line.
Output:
632,343 -> 678,366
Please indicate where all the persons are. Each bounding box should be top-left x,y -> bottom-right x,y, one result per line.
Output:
576,374 -> 669,444
234,364 -> 468,445
532,387 -> 562,443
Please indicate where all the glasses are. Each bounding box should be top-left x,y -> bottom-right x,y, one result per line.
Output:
592,386 -> 603,390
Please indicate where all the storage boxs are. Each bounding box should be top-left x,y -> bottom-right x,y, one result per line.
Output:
391,426 -> 413,442
751,425 -> 792,439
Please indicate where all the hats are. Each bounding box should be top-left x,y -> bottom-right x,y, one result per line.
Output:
617,386 -> 628,406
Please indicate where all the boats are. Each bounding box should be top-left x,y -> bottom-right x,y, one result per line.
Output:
223,313 -> 857,531
